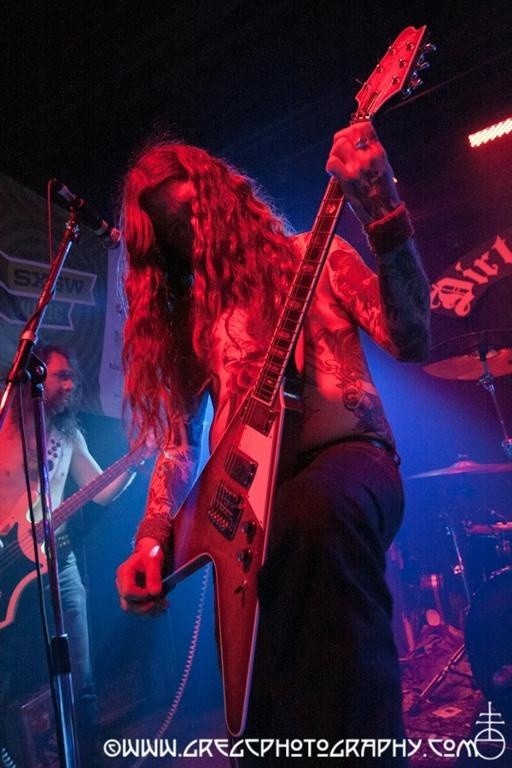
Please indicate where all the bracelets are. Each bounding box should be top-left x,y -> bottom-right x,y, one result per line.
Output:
128,458 -> 144,474
364,204 -> 413,252
132,514 -> 170,551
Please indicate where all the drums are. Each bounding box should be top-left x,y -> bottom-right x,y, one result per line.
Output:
465,567 -> 511,726
466,523 -> 511,565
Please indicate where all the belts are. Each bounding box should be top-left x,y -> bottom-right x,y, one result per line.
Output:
56,533 -> 71,550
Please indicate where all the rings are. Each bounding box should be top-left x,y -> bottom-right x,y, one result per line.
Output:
354,137 -> 369,149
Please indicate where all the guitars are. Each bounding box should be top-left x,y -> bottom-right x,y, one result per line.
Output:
136,27 -> 438,738
0,427 -> 158,629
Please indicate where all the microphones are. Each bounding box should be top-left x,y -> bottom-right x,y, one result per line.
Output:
56,184 -> 122,249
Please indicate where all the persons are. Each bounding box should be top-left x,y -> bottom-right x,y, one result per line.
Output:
114,121 -> 431,766
0,343 -> 160,768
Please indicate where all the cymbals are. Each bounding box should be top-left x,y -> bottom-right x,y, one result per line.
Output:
426,349 -> 511,380
402,459 -> 510,484
